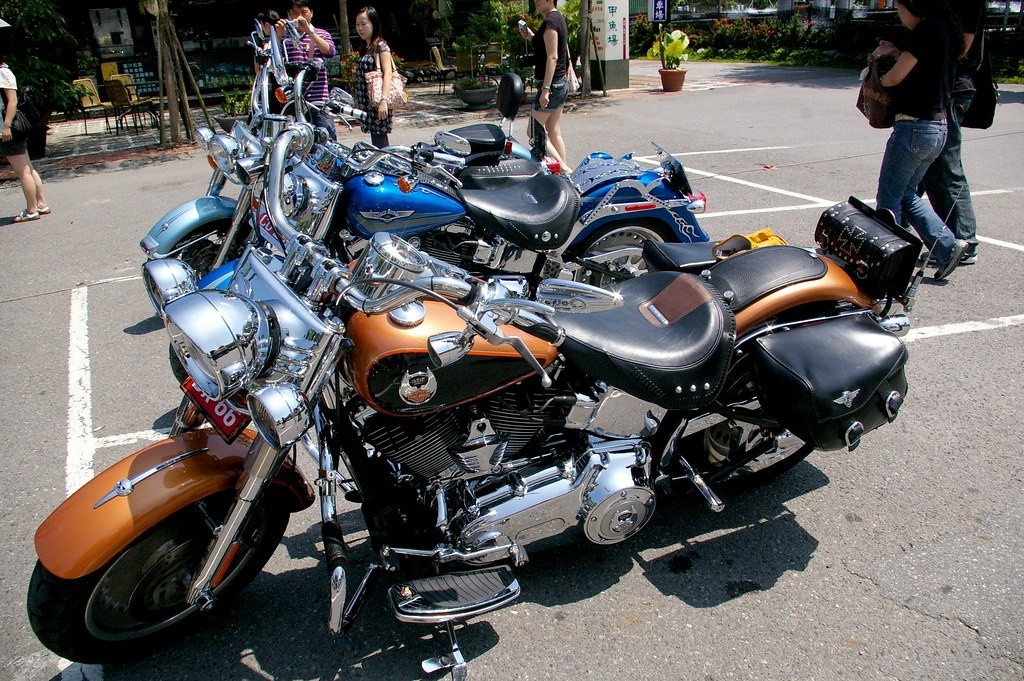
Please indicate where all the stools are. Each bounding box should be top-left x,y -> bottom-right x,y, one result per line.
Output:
429,42 -> 444,64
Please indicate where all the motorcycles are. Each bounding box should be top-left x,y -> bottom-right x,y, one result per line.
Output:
26,8 -> 922,681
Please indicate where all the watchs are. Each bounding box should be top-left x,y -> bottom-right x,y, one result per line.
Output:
381,96 -> 387,99
310,30 -> 314,36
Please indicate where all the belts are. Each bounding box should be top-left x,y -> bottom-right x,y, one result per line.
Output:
894,114 -> 918,121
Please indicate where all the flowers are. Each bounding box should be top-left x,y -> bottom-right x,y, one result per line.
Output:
451,75 -> 494,98
339,51 -> 407,91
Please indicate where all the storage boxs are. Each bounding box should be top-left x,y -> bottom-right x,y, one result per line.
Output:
642,239 -> 721,274
813,194 -> 925,298
744,310 -> 912,451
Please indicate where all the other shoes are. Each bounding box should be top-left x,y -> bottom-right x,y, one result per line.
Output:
37,206 -> 52,215
13,210 -> 40,222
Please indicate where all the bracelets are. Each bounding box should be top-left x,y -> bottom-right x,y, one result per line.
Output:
541,86 -> 550,90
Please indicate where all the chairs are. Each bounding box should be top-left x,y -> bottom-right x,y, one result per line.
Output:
72,74 -> 159,136
432,47 -> 459,96
479,43 -> 504,82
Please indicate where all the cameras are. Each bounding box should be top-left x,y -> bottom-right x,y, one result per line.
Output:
518,20 -> 527,31
287,20 -> 299,27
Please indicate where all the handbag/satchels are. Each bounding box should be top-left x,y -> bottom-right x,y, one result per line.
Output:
856,41 -> 902,129
364,39 -> 408,109
566,61 -> 579,96
3,91 -> 47,137
961,35 -> 997,129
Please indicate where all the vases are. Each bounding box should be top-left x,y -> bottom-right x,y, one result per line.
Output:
457,85 -> 497,111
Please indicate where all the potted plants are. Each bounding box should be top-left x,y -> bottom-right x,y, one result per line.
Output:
424,21 -> 441,43
507,66 -> 535,105
213,86 -> 253,133
647,29 -> 690,92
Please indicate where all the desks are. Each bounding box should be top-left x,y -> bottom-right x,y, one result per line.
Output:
98,80 -> 166,136
458,43 -> 486,77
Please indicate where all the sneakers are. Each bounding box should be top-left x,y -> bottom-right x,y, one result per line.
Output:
934,240 -> 969,281
921,246 -> 977,264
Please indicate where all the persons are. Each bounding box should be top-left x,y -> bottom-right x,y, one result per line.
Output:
867,0 -> 990,282
246,0 -> 337,143
354,6 -> 396,150
0,55 -> 52,223
519,0 -> 573,175
